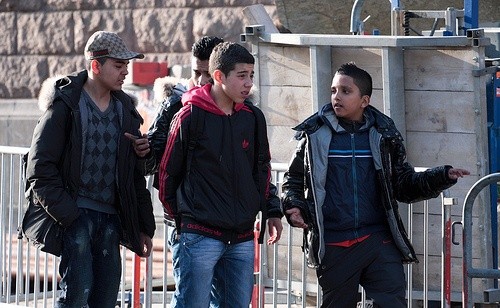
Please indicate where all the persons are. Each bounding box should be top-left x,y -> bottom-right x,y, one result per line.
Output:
124,36 -> 284,245
159,41 -> 271,308
26,31 -> 156,308
280,63 -> 472,308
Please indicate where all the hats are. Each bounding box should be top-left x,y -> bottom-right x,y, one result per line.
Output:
84,31 -> 144,61
